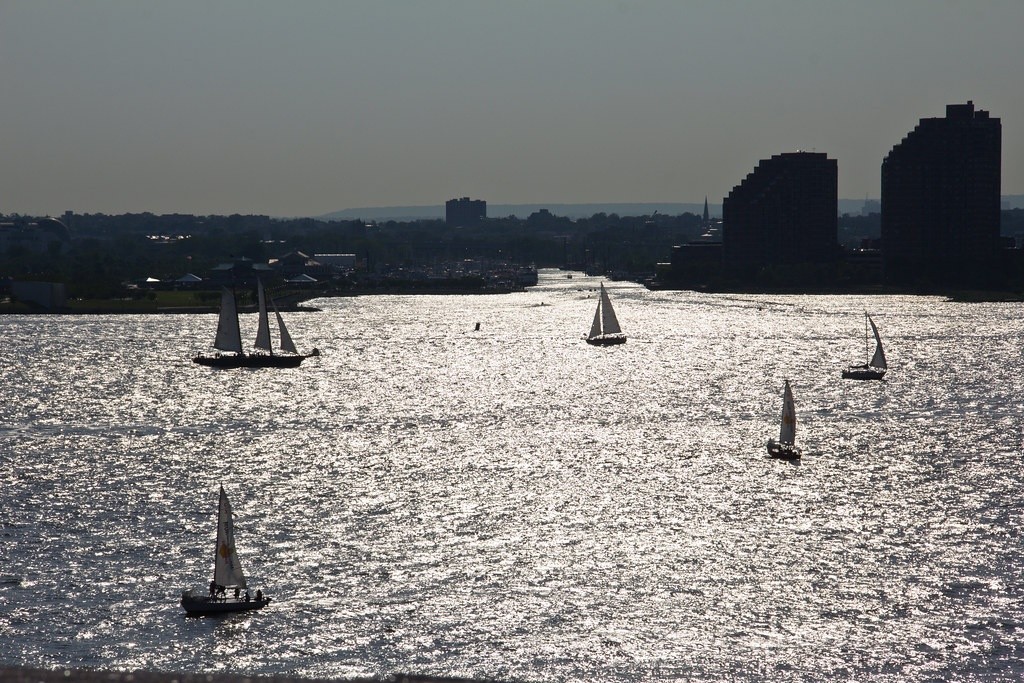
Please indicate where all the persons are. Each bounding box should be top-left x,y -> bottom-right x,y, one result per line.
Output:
255,589 -> 262,601
195,350 -> 267,361
216,585 -> 226,598
234,585 -> 240,600
208,581 -> 216,600
245,590 -> 252,603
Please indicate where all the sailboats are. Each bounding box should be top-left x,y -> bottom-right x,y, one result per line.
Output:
767,380 -> 802,462
192,291 -> 250,369
249,276 -> 317,368
586,281 -> 627,347
839,312 -> 888,382
180,483 -> 272,617
